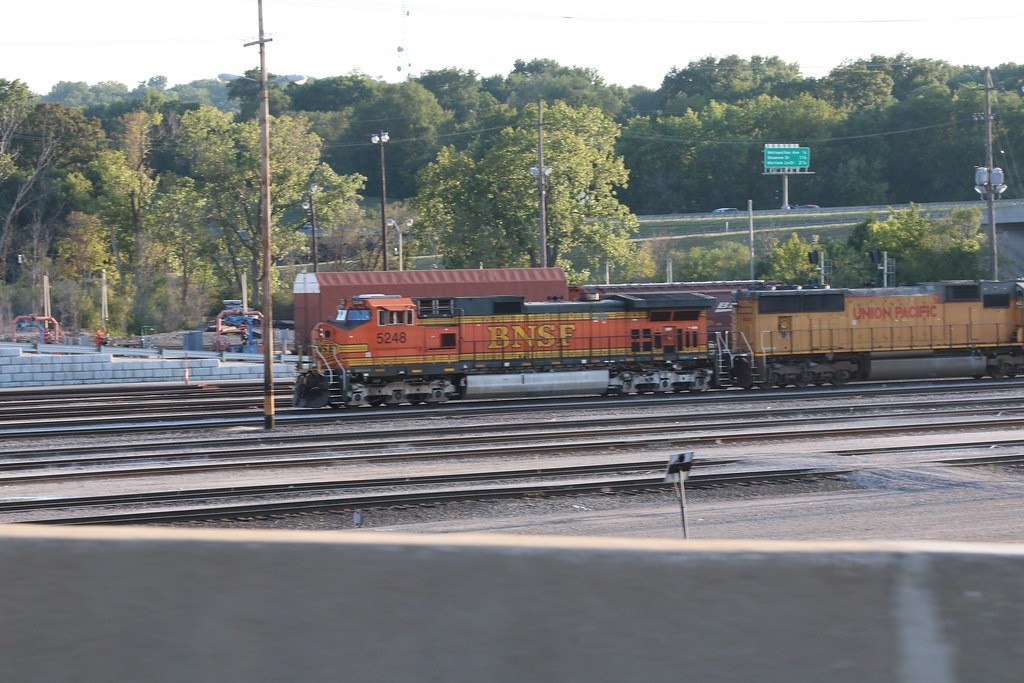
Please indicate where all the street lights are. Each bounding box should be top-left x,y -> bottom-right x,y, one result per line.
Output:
369,125 -> 394,269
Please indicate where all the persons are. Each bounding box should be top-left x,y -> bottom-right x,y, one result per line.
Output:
96,327 -> 104,346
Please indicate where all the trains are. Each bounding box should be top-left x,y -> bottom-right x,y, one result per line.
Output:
292,267 -> 1024,413
